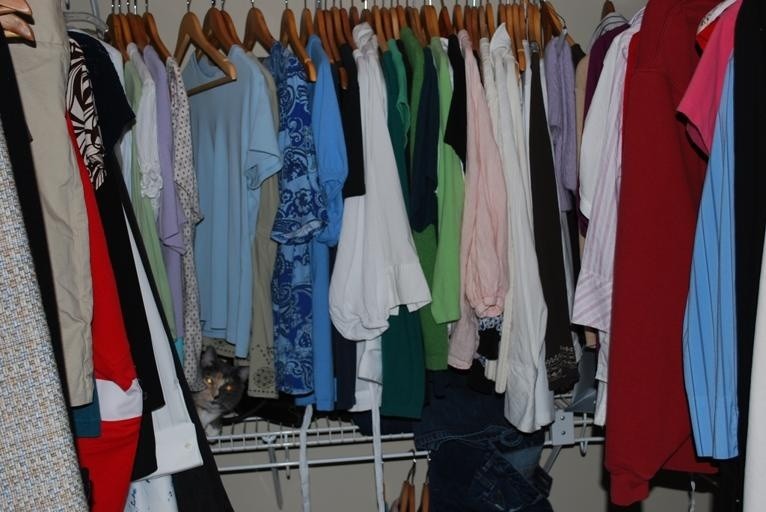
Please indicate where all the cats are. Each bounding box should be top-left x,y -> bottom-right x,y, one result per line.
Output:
188,345 -> 250,432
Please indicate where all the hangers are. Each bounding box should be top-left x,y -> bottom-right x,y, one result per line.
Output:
58,0 -> 614,96
397,449 -> 433,512
